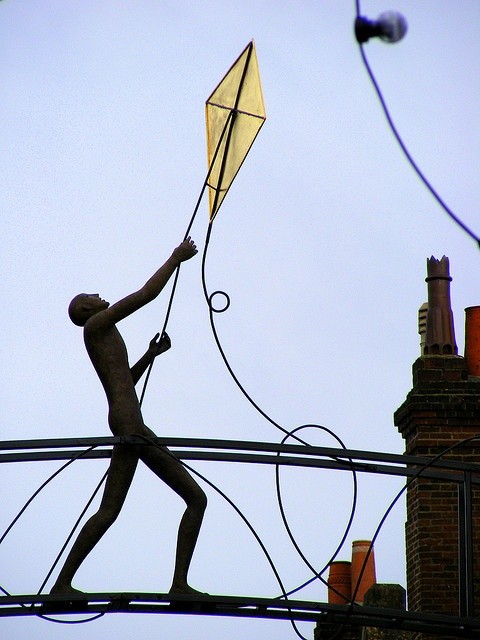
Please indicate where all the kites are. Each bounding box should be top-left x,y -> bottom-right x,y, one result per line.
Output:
205,40 -> 267,223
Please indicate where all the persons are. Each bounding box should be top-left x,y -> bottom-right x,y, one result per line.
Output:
49,239 -> 207,602
